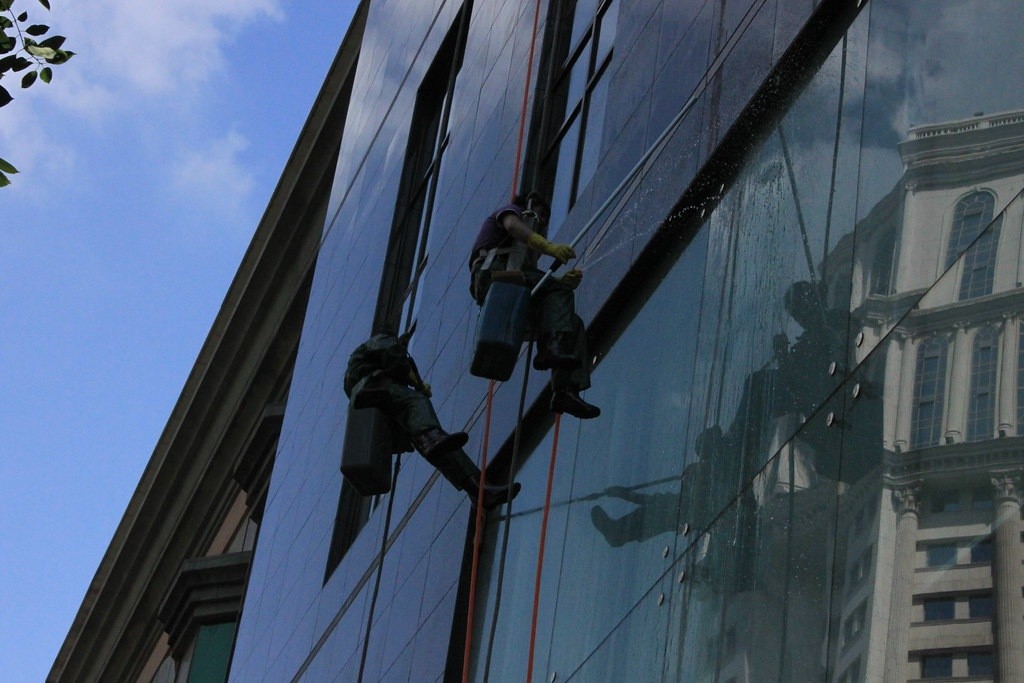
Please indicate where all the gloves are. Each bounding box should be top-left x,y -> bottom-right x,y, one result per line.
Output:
407,370 -> 432,396
528,232 -> 576,265
552,268 -> 584,292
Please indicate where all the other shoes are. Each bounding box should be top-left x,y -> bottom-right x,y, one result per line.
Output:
549,390 -> 601,420
532,347 -> 584,371
481,483 -> 521,511
591,505 -> 626,546
424,432 -> 469,457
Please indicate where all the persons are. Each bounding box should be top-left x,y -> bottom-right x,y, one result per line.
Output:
341,332 -> 521,510
589,281 -> 886,553
468,195 -> 601,420
